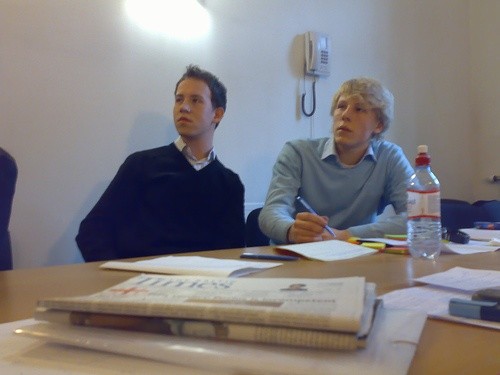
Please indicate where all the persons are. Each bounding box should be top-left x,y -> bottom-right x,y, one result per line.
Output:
259,78 -> 421,247
74,68 -> 248,264
0,147 -> 19,272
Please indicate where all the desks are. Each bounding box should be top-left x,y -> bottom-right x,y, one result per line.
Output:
0,246 -> 500,375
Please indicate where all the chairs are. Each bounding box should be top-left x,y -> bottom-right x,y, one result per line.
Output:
439,199 -> 500,239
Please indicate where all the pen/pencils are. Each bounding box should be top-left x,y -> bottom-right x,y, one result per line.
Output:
240,253 -> 297,261
296,196 -> 337,238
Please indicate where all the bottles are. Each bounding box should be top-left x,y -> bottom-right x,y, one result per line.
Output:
407,145 -> 441,259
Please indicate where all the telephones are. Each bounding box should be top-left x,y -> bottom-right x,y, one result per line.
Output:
300,31 -> 330,117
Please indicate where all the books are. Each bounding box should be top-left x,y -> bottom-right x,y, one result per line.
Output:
34,274 -> 387,350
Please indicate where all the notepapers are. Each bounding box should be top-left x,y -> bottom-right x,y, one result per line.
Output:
361,242 -> 386,250
